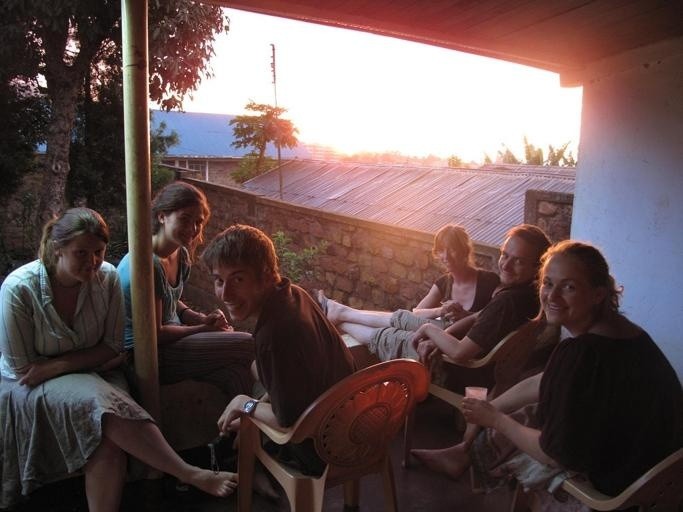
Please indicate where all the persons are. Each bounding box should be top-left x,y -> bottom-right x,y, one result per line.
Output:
409,239 -> 682,495
412,226 -> 501,319
0,207 -> 239,512
114,182 -> 258,401
202,225 -> 358,501
312,224 -> 551,396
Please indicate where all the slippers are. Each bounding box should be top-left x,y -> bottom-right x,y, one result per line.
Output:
313,288 -> 328,316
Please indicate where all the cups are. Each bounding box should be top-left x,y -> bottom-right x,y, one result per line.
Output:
465,386 -> 488,400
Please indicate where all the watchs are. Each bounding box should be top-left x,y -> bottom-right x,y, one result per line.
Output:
241,398 -> 259,417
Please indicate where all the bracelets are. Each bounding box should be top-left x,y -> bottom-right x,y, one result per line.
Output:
181,307 -> 192,319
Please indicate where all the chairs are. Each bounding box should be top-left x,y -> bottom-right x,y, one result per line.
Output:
235,358 -> 430,512
402,318 -> 548,469
511,448 -> 682,511
